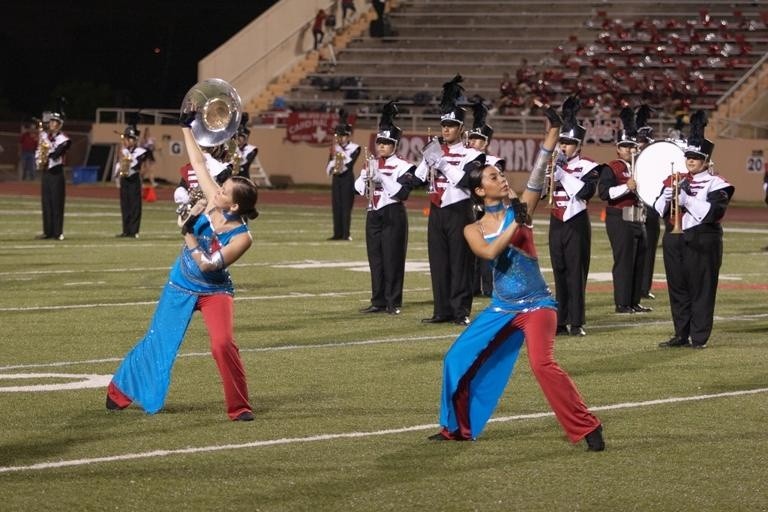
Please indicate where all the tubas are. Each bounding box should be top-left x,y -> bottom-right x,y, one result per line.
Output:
175,77 -> 243,228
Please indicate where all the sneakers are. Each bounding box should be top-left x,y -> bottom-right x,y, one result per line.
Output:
105,394 -> 122,409
429,432 -> 464,442
584,423 -> 605,452
238,411 -> 255,421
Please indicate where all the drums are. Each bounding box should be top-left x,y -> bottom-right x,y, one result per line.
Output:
631,137 -> 688,208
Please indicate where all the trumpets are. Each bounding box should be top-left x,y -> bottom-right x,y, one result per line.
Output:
668,162 -> 685,235
34,118 -> 51,170
543,148 -> 560,210
426,126 -> 439,195
332,131 -> 344,174
363,146 -> 378,213
113,129 -> 133,178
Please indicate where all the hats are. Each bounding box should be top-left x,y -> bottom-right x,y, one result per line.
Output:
438,72 -> 468,127
558,88 -> 587,145
239,112 -> 251,138
468,93 -> 494,143
332,107 -> 353,136
125,112 -> 142,139
635,103 -> 654,144
49,95 -> 67,123
614,106 -> 635,147
375,99 -> 403,144
683,108 -> 715,163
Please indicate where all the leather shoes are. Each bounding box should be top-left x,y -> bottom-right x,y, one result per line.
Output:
421,314 -> 452,323
632,303 -> 653,312
116,233 -> 127,238
658,336 -> 690,348
360,305 -> 386,314
386,305 -> 401,315
642,291 -> 656,299
56,233 -> 65,240
570,326 -> 587,337
131,233 -> 139,238
35,232 -> 48,239
453,315 -> 471,326
614,304 -> 636,314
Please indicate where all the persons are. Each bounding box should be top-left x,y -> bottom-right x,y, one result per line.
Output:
106,112 -> 260,421
598,105 -> 653,313
638,105 -> 660,299
231,112 -> 259,226
326,108 -> 361,241
420,75 -> 486,326
177,144 -> 240,228
358,99 -> 416,315
139,127 -> 159,189
311,0 -> 356,49
653,110 -> 735,348
35,102 -> 71,240
427,99 -> 606,452
20,120 -> 37,181
489,9 -> 767,125
545,94 -> 600,338
115,115 -> 153,238
465,93 -> 506,298
